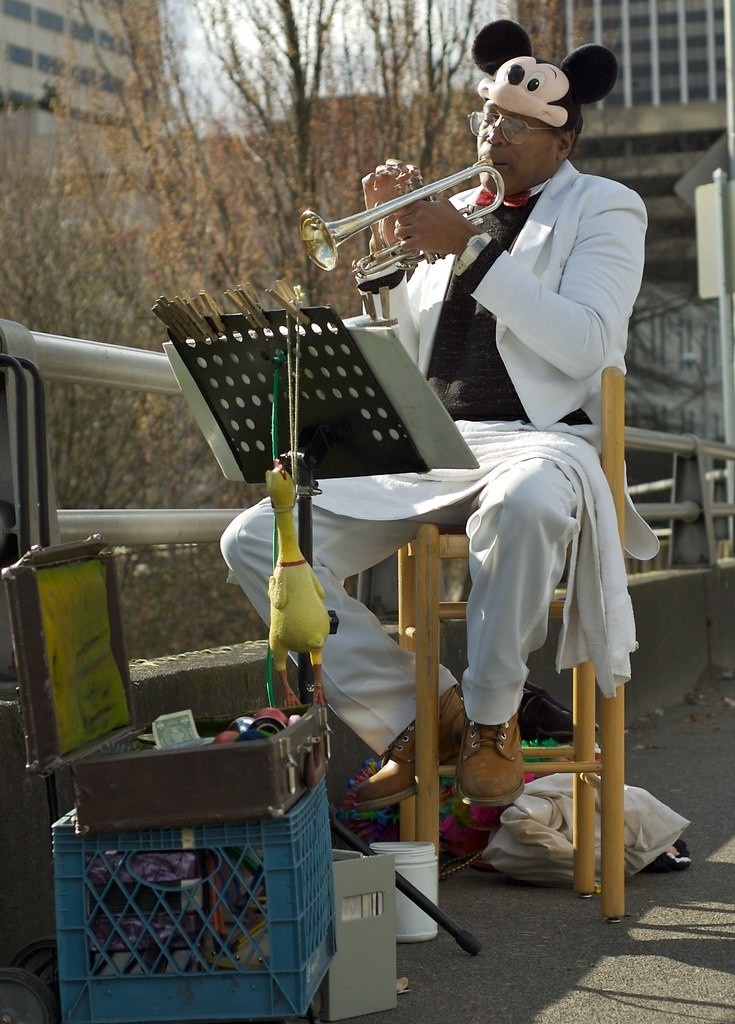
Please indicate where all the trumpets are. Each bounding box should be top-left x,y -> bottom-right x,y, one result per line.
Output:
297,150 -> 506,287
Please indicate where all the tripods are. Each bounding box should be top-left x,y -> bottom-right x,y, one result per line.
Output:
170,305 -> 483,956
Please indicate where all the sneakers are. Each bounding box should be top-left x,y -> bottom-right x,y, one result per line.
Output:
355,684 -> 466,812
456,708 -> 525,804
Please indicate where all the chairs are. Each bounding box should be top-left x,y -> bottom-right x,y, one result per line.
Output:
397,364 -> 629,921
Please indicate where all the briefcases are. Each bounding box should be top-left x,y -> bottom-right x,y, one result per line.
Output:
0,535 -> 330,835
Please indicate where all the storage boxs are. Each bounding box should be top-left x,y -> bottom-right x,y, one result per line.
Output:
0,532 -> 333,833
49,774 -> 339,1024
320,849 -> 398,1024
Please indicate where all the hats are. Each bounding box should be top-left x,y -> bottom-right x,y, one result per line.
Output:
472,19 -> 618,132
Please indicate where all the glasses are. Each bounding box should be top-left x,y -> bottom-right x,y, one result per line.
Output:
467,110 -> 554,145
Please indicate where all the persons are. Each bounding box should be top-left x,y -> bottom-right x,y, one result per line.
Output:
220,19 -> 648,808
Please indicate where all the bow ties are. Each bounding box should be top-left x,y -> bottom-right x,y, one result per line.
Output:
476,189 -> 531,207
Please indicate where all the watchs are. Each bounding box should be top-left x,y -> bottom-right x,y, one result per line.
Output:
452,232 -> 492,276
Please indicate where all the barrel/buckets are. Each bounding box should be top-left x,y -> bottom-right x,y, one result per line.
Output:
370,842 -> 439,943
